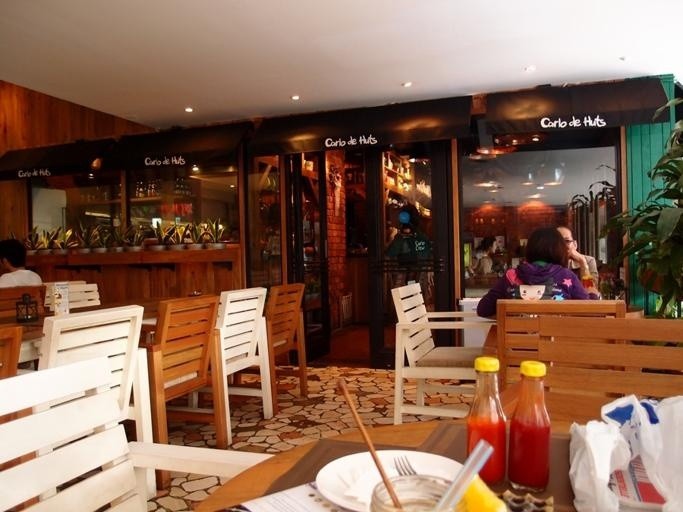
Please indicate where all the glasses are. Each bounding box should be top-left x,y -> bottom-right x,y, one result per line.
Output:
566,239 -> 575,244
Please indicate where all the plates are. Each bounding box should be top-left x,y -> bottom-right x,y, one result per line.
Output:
314,450 -> 464,512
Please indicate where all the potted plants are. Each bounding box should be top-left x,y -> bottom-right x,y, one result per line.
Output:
7,217 -> 227,253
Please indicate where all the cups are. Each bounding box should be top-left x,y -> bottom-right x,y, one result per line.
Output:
369,473 -> 468,512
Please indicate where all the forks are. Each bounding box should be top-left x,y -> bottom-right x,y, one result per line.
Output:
389,454 -> 418,475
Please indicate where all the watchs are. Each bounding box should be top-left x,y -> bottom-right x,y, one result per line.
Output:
469,272 -> 475,277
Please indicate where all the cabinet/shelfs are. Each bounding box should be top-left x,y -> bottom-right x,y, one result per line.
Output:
254,154 -> 319,364
365,150 -> 431,359
78,194 -> 197,239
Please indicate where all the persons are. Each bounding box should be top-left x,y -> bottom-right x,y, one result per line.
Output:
464,235 -> 497,279
257,202 -> 280,286
475,225 -> 604,368
491,239 -> 511,276
0,238 -> 42,288
384,202 -> 433,287
556,227 -> 600,294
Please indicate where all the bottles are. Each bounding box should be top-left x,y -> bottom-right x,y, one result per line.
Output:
466,357 -> 507,483
508,359 -> 551,489
134,178 -> 191,197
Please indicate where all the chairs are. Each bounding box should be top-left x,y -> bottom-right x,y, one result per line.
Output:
390,280 -> 683,426
0,284 -> 309,512
